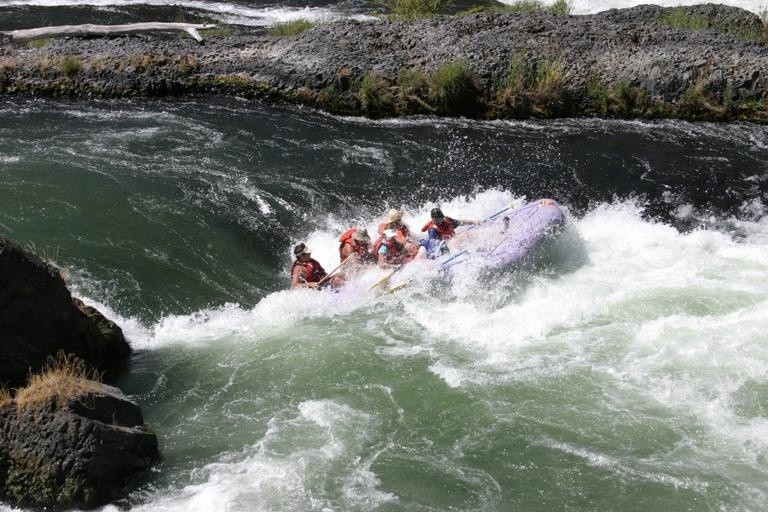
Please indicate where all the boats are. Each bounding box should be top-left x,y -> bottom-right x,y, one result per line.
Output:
328,196 -> 565,305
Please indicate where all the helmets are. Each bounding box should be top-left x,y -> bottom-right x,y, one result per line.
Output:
431,207 -> 443,217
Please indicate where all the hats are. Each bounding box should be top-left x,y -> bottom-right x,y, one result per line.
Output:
351,227 -> 371,241
294,246 -> 313,257
383,209 -> 402,224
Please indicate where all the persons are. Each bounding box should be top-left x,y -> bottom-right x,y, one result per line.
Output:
368,227 -> 420,272
288,240 -> 350,292
372,207 -> 411,234
417,207 -> 483,258
339,227 -> 377,278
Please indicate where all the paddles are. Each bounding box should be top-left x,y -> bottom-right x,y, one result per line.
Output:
367,243 -> 474,297
448,194 -> 528,242
